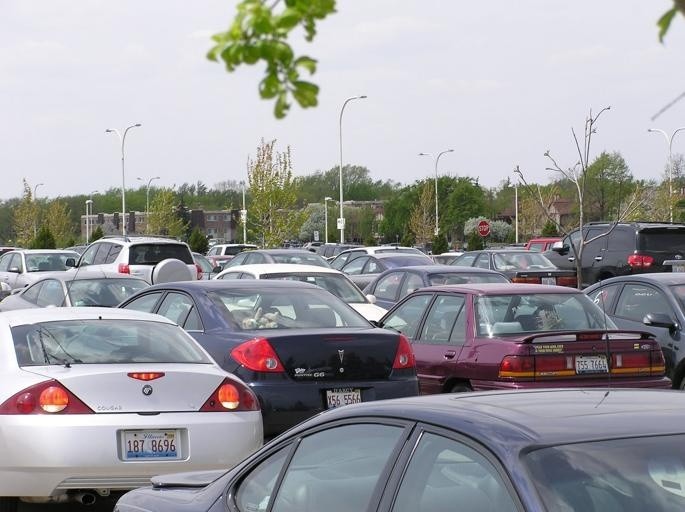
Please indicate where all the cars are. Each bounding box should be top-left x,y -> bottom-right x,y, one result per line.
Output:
105,380 -> 684,512
1,299 -> 271,500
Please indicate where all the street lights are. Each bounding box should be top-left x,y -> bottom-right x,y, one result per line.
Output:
105,119 -> 143,234
136,175 -> 162,233
86,198 -> 95,245
647,126 -> 685,222
338,94 -> 368,244
33,181 -> 45,238
90,188 -> 100,239
417,148 -> 456,237
237,178 -> 250,245
324,194 -> 333,244
